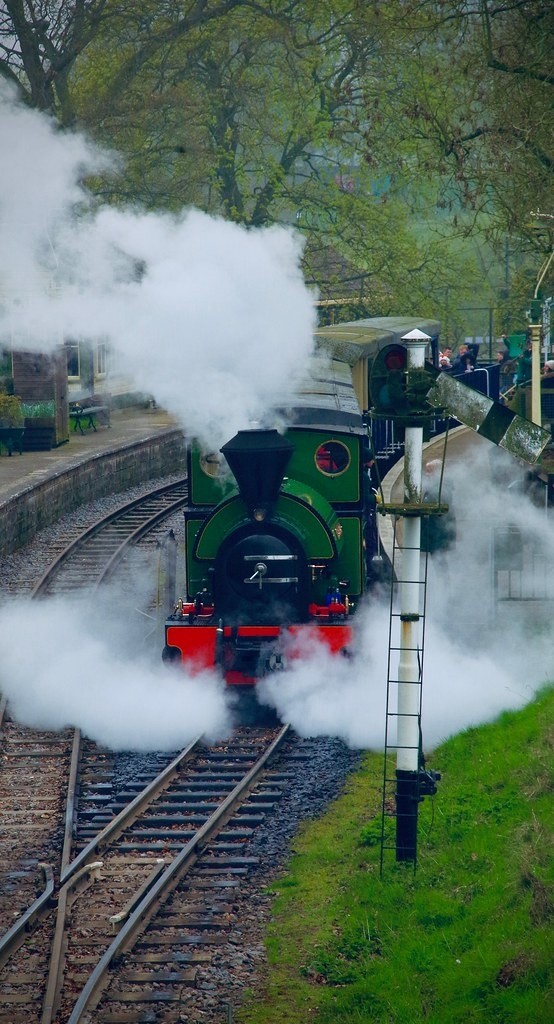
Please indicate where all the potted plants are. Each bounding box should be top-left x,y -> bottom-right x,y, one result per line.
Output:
0,390 -> 27,456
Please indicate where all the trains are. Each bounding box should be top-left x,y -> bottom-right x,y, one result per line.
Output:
162,312 -> 502,688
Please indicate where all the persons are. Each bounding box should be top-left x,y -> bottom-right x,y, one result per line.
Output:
455,343 -> 479,373
363,448 -> 375,471
543,360 -> 554,374
499,383 -> 514,404
519,342 -> 532,365
497,335 -> 510,363
439,347 -> 452,368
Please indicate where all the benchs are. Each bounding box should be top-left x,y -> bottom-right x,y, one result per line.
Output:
69,389 -> 107,436
